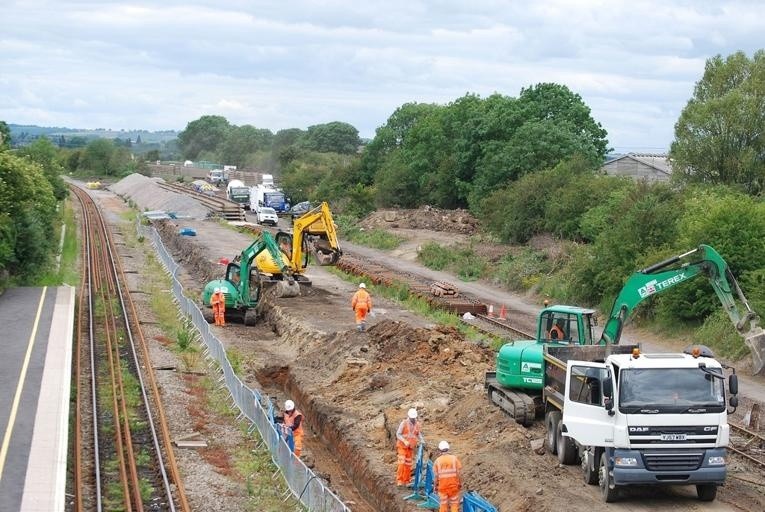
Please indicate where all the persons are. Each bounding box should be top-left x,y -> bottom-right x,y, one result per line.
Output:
549,317 -> 566,340
282,399 -> 304,465
394,408 -> 425,487
433,441 -> 462,512
210,288 -> 226,326
351,282 -> 372,332
280,237 -> 291,259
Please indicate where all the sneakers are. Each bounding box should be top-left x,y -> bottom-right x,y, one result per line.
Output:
397,480 -> 413,488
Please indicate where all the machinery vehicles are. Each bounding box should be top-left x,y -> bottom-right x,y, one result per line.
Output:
483,245 -> 765,426
200,202 -> 340,326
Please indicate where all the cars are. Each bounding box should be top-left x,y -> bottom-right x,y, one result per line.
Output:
256,206 -> 279,227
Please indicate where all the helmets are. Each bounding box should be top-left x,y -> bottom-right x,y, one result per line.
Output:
439,441 -> 449,451
360,282 -> 366,288
285,400 -> 294,411
214,288 -> 219,292
408,409 -> 418,419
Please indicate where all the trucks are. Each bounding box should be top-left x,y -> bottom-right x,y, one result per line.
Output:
209,164 -> 284,214
542,343 -> 739,501
